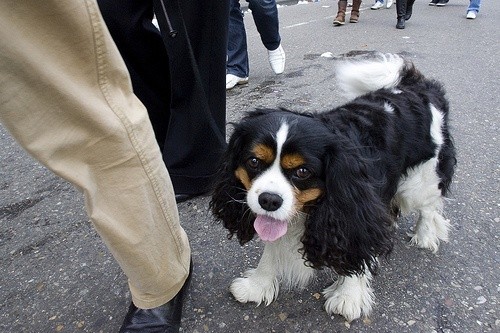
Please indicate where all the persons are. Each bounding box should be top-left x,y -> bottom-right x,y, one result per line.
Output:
396,0 -> 415,28
0,0 -> 192,333
333,0 -> 362,25
466,0 -> 480,19
97,1 -> 226,202
428,0 -> 449,6
370,0 -> 393,9
226,0 -> 287,89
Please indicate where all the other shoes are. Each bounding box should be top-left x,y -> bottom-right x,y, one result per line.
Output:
226,72 -> 250,89
386,0 -> 393,8
436,0 -> 450,5
174,192 -> 201,202
466,10 -> 477,18
428,0 -> 442,5
370,1 -> 384,9
267,42 -> 286,74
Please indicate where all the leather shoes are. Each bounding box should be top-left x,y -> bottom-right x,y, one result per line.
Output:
396,15 -> 405,28
406,4 -> 413,20
118,252 -> 193,333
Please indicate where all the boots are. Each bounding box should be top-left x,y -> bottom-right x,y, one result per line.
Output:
350,0 -> 362,22
333,0 -> 347,24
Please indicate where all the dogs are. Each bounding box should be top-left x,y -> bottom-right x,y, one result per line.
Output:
208,51 -> 458,321
